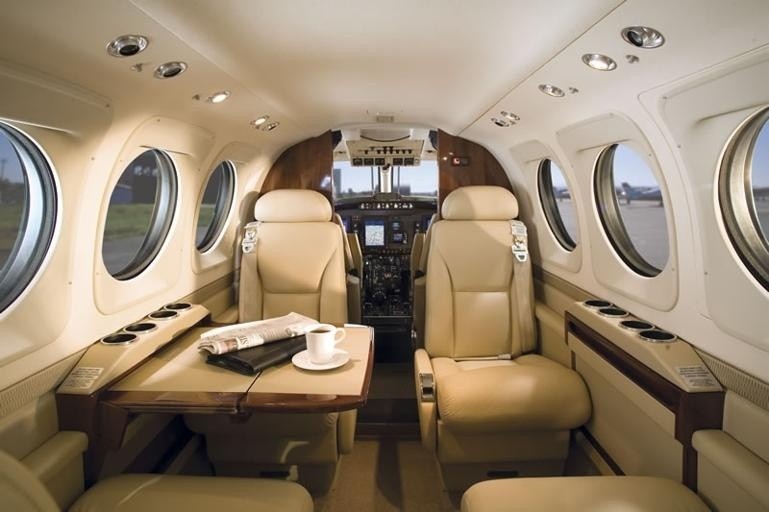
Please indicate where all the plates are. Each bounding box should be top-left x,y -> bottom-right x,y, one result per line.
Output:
291,347 -> 351,372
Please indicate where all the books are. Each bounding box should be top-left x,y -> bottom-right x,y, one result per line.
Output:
205,334 -> 307,375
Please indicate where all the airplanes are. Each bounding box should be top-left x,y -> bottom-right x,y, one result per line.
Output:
550,180 -> 663,205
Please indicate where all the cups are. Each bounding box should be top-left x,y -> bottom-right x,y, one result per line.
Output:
304,323 -> 347,365
